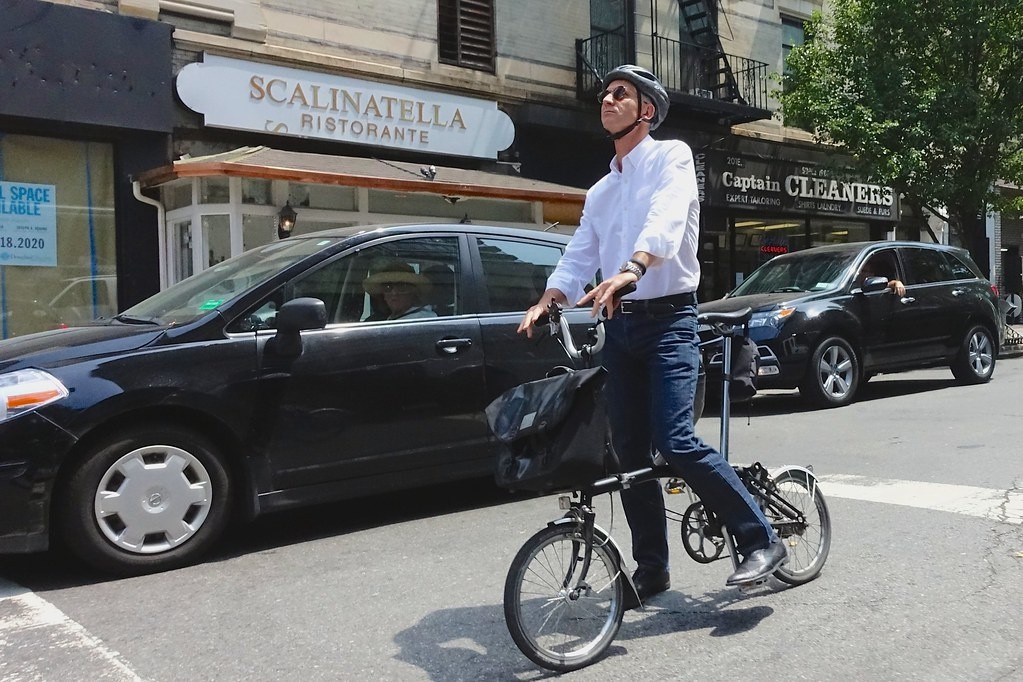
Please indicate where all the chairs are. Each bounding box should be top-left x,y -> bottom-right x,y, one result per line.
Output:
362,294 -> 390,322
418,266 -> 455,318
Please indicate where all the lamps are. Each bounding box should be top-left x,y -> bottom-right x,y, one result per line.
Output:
277,200 -> 298,240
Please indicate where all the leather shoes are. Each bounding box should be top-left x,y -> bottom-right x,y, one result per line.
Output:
623,569 -> 670,610
725,539 -> 787,585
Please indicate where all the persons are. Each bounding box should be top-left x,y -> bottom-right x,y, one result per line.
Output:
517,64 -> 789,612
361,263 -> 436,322
856,260 -> 906,297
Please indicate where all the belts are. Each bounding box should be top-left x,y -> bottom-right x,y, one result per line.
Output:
602,292 -> 696,314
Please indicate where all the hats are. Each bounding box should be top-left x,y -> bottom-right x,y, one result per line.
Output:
361,262 -> 431,301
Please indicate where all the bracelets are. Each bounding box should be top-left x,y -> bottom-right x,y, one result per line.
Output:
627,257 -> 646,276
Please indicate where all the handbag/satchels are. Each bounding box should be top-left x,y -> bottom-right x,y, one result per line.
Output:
730,325 -> 760,401
483,365 -> 609,489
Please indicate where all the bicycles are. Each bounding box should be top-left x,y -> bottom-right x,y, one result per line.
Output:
503,282 -> 835,672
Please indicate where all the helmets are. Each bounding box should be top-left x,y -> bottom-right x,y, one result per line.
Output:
601,64 -> 669,130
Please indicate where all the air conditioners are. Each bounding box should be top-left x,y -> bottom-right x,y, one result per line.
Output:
688,88 -> 713,100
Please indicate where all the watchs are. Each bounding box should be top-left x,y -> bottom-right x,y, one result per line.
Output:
619,260 -> 642,281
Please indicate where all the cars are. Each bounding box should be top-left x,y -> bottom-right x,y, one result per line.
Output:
0,274 -> 119,341
694,240 -> 1006,410
0,219 -> 706,577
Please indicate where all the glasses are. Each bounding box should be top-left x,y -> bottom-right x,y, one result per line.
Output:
597,85 -> 649,104
383,283 -> 410,293
